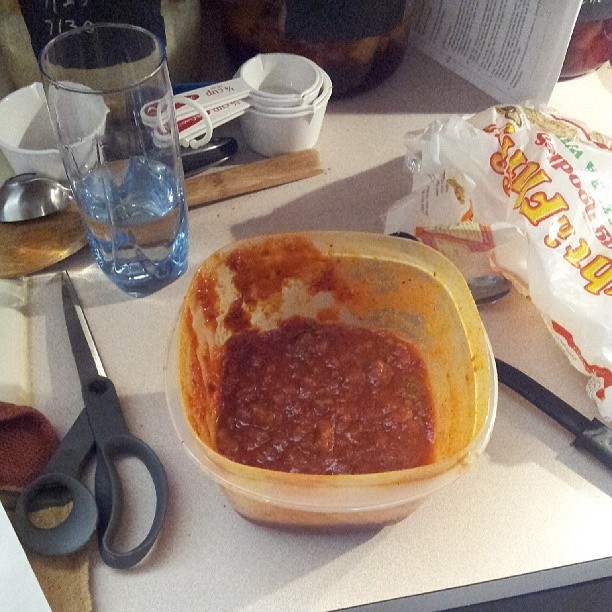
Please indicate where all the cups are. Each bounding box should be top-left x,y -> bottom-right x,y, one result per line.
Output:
36,22 -> 190,299
0,79 -> 111,183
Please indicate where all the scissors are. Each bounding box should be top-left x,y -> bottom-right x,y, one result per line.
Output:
12,269 -> 169,569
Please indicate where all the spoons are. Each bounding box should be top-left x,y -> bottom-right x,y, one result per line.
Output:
378,233 -> 611,478
0,136 -> 238,223
